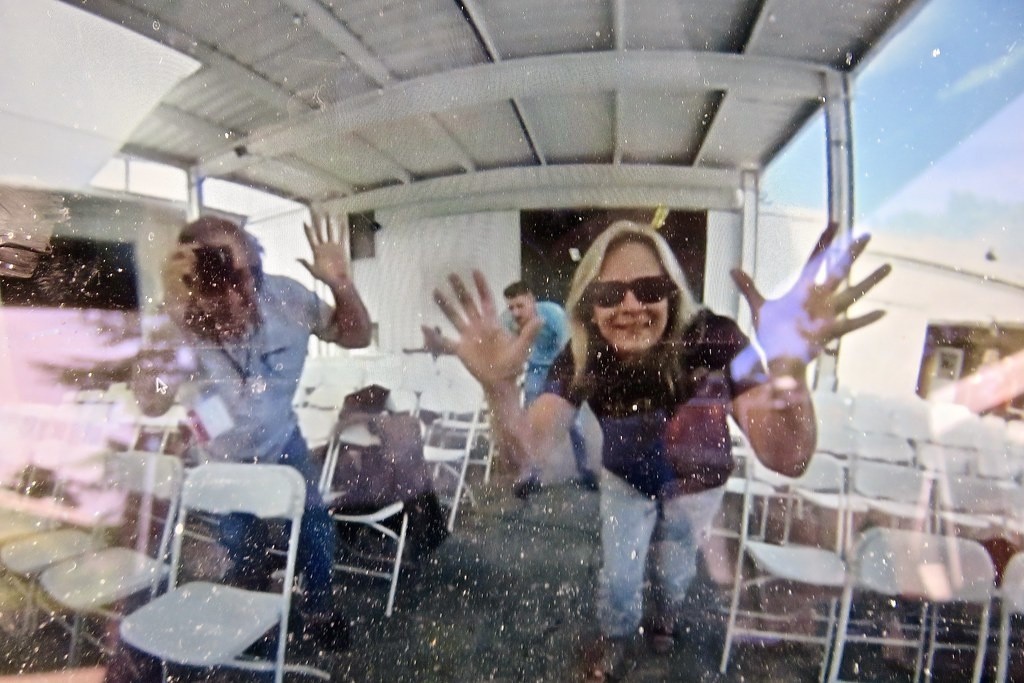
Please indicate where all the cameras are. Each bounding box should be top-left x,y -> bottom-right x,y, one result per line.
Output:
182,245 -> 232,296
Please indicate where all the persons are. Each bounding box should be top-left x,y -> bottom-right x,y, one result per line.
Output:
421,220 -> 892,682
131,209 -> 373,662
498,279 -> 600,498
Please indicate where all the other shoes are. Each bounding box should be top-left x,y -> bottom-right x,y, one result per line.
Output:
586,640 -> 642,683
648,609 -> 680,661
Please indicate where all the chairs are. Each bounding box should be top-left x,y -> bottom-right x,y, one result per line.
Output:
0,351 -> 527,683
702,388 -> 1024,683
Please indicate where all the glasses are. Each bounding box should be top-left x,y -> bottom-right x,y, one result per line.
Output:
587,275 -> 680,310
